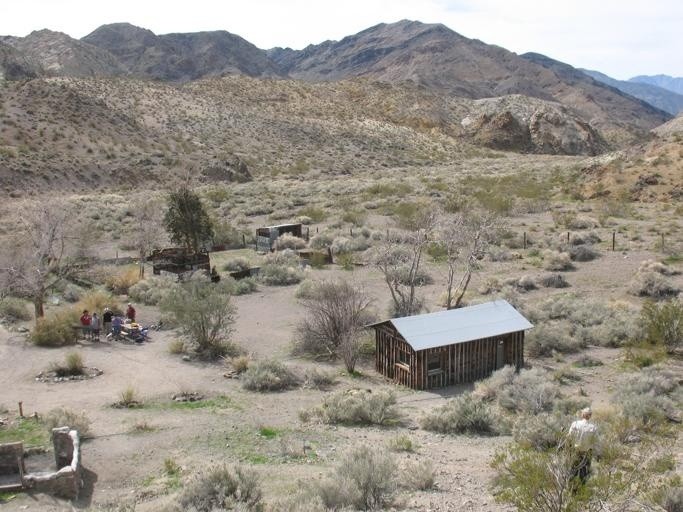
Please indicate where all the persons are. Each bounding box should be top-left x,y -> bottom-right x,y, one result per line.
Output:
566,404 -> 596,494
91,313 -> 101,340
102,307 -> 113,336
126,303 -> 135,322
112,314 -> 123,341
80,310 -> 92,340
